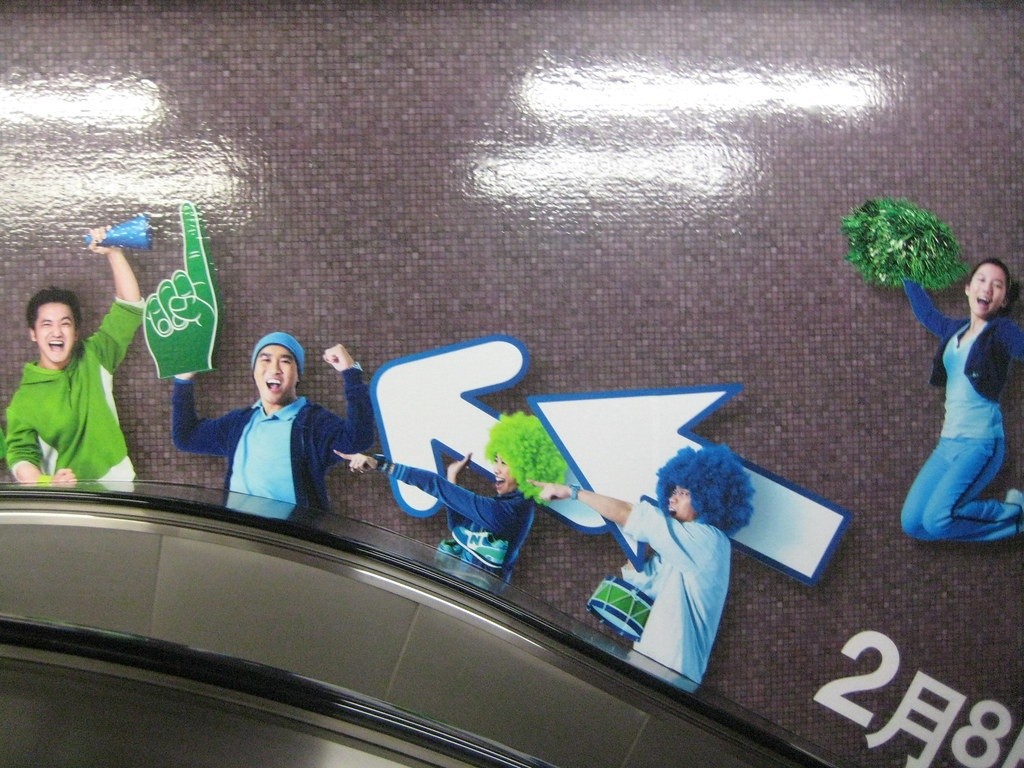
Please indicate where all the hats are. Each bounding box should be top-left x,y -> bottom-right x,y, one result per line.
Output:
250,332 -> 303,376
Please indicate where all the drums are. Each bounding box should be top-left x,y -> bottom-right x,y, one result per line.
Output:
587,575 -> 653,642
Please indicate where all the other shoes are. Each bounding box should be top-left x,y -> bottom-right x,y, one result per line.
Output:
1004,488 -> 1024,533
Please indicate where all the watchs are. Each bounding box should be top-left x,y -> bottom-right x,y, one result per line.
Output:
372,453 -> 386,472
568,483 -> 580,500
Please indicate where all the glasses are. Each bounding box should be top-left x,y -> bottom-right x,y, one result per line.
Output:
672,488 -> 691,497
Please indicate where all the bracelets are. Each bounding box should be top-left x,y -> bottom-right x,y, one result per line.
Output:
36,474 -> 53,490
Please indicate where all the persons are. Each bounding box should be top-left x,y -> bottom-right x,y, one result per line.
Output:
332,409 -> 570,596
171,332 -> 377,528
5,224 -> 147,494
528,444 -> 756,695
900,257 -> 1024,544
